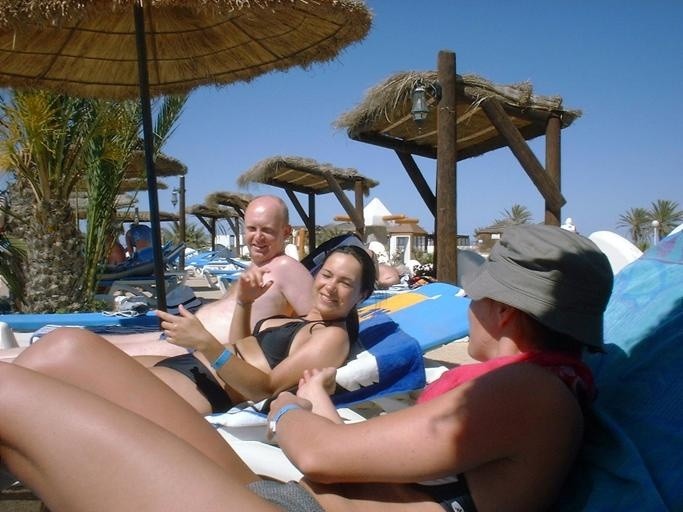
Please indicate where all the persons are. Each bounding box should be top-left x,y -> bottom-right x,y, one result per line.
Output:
125,224 -> 152,255
1,195 -> 319,366
374,262 -> 412,290
106,233 -> 127,264
125,244 -> 376,417
1,227 -> 614,512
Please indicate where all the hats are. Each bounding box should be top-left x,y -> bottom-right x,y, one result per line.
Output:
165,283 -> 201,315
457,223 -> 614,354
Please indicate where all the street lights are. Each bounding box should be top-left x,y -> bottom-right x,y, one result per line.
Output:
171,176 -> 185,271
651,221 -> 660,245
410,50 -> 456,284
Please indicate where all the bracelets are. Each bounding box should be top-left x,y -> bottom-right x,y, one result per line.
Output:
273,403 -> 300,422
211,348 -> 233,369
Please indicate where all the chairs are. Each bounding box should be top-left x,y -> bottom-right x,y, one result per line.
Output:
0,223 -> 683,512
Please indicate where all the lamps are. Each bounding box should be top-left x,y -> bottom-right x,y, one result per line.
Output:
411,80 -> 442,127
171,189 -> 181,207
